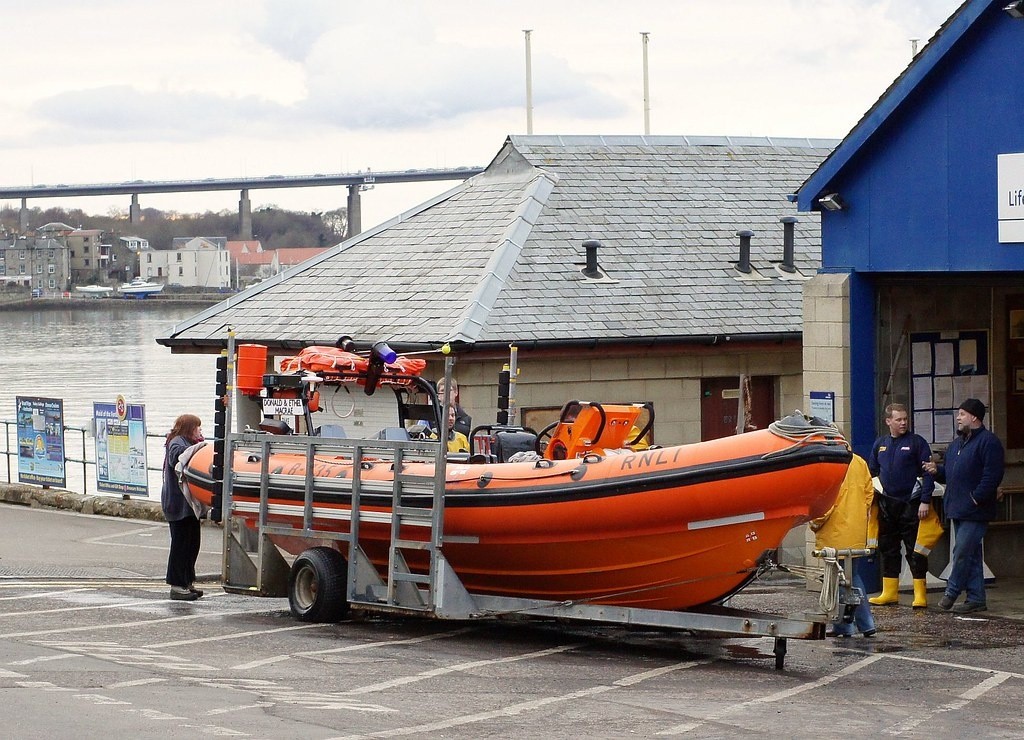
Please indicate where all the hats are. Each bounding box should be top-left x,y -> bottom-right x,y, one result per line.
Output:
959,398 -> 986,422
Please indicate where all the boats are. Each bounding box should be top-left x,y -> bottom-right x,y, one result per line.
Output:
118,276 -> 165,299
76,285 -> 114,294
181,398 -> 854,612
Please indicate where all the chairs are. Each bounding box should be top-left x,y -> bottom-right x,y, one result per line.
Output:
491,424 -> 541,463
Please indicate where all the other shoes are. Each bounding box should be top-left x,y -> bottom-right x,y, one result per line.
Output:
826,630 -> 850,638
169,588 -> 198,601
938,593 -> 957,609
864,629 -> 876,637
188,586 -> 203,598
958,603 -> 988,614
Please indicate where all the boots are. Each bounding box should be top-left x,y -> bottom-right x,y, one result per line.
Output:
869,577 -> 900,606
912,579 -> 927,608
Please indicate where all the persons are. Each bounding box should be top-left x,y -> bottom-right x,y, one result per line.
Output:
418,376 -> 472,453
869,404 -> 937,607
922,398 -> 1005,613
160,414 -> 211,600
809,451 -> 878,638
562,401 -> 648,451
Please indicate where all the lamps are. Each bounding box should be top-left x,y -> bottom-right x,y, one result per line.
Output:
818,192 -> 848,214
